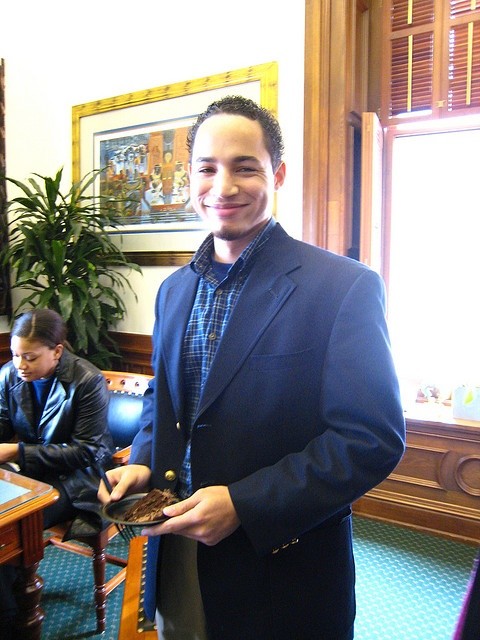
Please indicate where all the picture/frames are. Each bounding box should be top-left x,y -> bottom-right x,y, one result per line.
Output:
69,62 -> 279,268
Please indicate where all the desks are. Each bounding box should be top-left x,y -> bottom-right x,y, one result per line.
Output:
0,469 -> 62,640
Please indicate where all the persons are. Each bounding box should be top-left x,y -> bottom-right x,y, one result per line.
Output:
94,95 -> 408,639
0,307 -> 118,531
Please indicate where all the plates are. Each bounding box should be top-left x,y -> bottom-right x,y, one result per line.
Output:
102,493 -> 180,526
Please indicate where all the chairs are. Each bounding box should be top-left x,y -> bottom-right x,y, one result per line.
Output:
43,371 -> 156,634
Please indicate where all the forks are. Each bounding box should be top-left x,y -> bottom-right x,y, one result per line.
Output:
98,468 -> 137,542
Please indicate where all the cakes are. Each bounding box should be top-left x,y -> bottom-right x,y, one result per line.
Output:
123,490 -> 177,524
